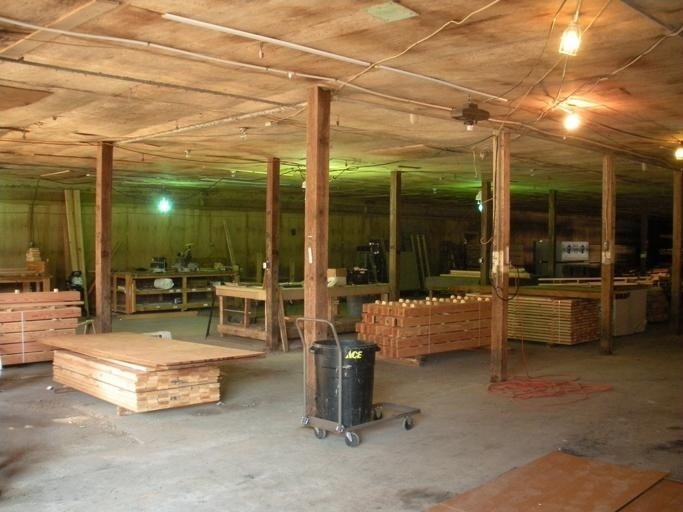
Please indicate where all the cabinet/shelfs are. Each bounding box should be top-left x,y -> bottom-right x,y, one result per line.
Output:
112,267 -> 242,313
212,276 -> 391,344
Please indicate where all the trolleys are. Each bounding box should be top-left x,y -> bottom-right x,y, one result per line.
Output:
295,317 -> 421,448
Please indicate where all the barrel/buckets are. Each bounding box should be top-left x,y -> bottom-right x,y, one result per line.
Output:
309,337 -> 381,427
357,245 -> 386,282
